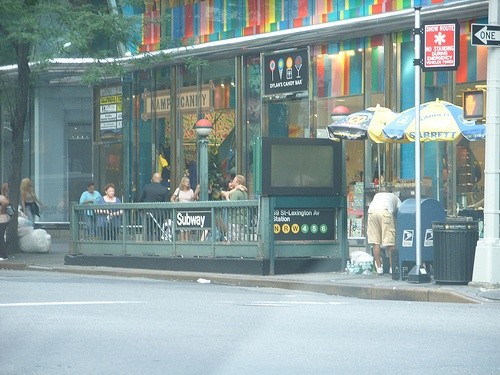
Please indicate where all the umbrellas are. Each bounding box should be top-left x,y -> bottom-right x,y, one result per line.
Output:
383,97 -> 487,201
326,103 -> 396,193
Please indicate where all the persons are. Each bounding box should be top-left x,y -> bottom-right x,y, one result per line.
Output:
367,192 -> 403,275
79,173 -> 249,242
0,177 -> 42,262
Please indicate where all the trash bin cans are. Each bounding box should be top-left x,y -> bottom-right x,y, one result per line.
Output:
396,198 -> 446,281
431,220 -> 478,283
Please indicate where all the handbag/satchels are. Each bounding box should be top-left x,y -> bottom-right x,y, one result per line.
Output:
0,195 -> 15,217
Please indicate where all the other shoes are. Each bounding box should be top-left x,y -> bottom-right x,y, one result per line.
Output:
375,257 -> 384,275
389,267 -> 392,274
0,256 -> 9,262
